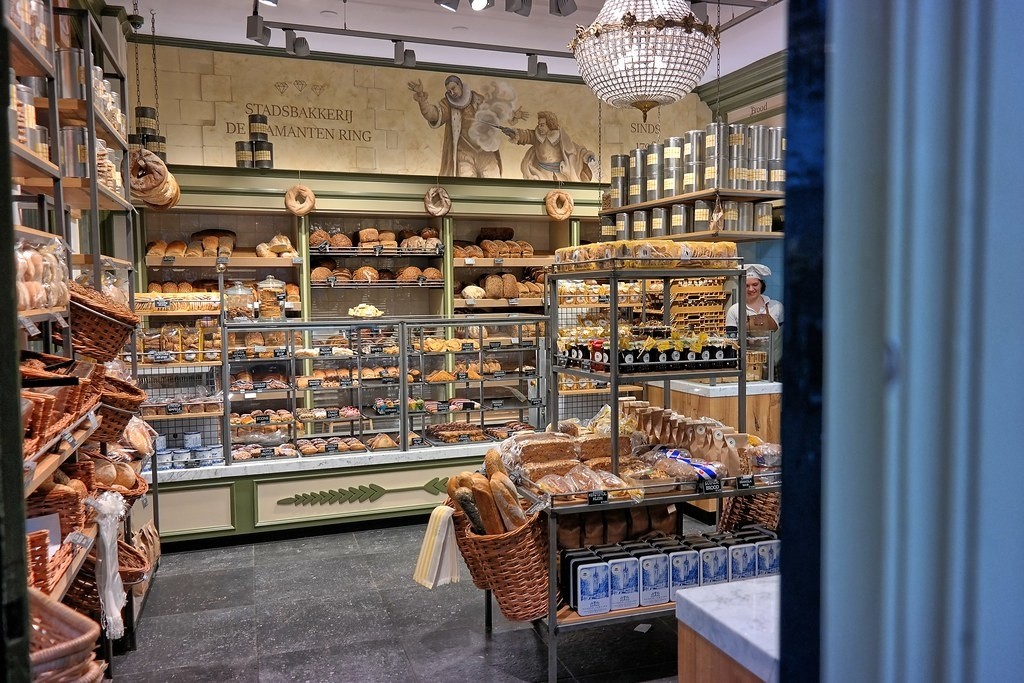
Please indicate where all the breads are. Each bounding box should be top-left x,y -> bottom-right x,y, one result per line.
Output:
147,227 -> 553,463
445,417 -> 726,534
284,186 -> 315,217
424,186 -> 452,217
120,150 -> 181,212
16,237 -> 155,492
546,190 -> 574,221
552,241 -> 739,304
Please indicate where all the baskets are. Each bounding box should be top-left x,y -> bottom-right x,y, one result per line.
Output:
442,497 -> 491,589
464,500 -> 562,622
89,376 -> 146,443
50,288 -> 139,361
22,382 -> 151,683
718,485 -> 782,533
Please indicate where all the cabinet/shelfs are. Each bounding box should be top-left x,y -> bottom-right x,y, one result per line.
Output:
485,267 -> 782,683
639,330 -> 781,449
53,6 -> 162,656
596,187 -> 784,242
100,163 -> 615,544
0,0 -> 276,683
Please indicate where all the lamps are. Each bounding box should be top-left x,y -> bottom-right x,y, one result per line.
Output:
255,27 -> 271,45
469,0 -> 495,12
246,14 -> 264,41
506,0 -> 521,12
566,0 -> 720,124
433,0 -> 459,12
404,50 -> 416,67
295,37 -> 310,56
286,30 -> 295,55
558,0 -> 578,16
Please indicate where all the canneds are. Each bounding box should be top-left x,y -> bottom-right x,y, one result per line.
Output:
143,431 -> 224,470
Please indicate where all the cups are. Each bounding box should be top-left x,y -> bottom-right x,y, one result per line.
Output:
634,207 -> 670,239
249,114 -> 267,142
236,141 -> 254,168
5,0 -> 126,197
255,141 -> 273,169
128,134 -> 141,150
601,216 -> 615,241
146,135 -> 166,164
630,144 -> 664,203
670,200 -> 775,237
135,107 -> 156,137
616,213 -> 633,241
664,124 -> 786,197
611,155 -> 630,208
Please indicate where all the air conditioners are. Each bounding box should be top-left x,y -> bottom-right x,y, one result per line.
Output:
394,42 -> 404,64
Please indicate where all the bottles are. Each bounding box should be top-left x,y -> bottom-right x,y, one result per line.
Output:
602,189 -> 611,211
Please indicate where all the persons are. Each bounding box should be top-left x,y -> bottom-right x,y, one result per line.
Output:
724,264 -> 784,382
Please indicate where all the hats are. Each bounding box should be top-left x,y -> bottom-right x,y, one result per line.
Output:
733,263 -> 771,281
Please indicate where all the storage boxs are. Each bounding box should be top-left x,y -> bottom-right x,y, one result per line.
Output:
22,279 -> 151,683
560,528 -> 781,617
